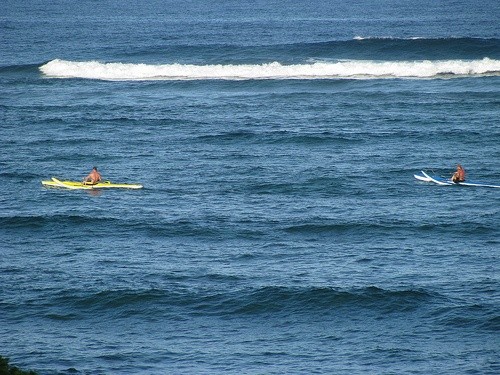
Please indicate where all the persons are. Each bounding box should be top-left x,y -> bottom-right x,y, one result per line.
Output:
82,167 -> 101,184
451,165 -> 464,183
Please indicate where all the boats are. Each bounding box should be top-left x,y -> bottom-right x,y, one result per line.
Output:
43,176 -> 142,191
414,169 -> 500,190
